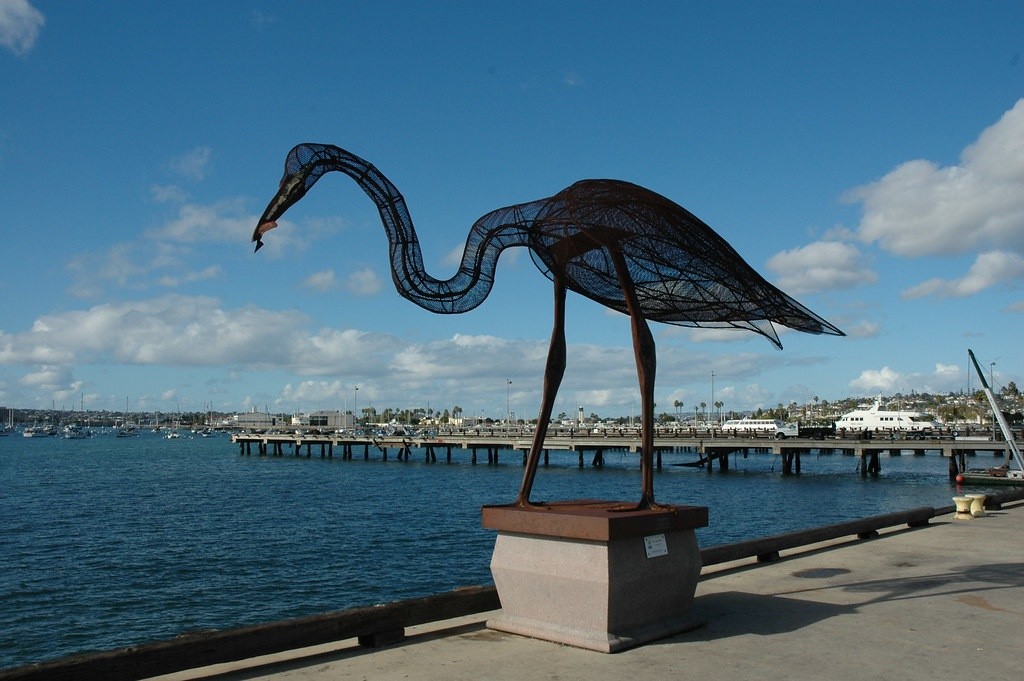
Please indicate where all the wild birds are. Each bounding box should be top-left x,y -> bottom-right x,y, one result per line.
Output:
251,142 -> 849,515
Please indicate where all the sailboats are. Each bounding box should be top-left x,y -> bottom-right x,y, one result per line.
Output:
0,387 -> 726,441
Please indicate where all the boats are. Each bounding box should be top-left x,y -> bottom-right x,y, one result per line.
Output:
826,400 -> 957,441
953,348 -> 1024,487
721,418 -> 798,441
